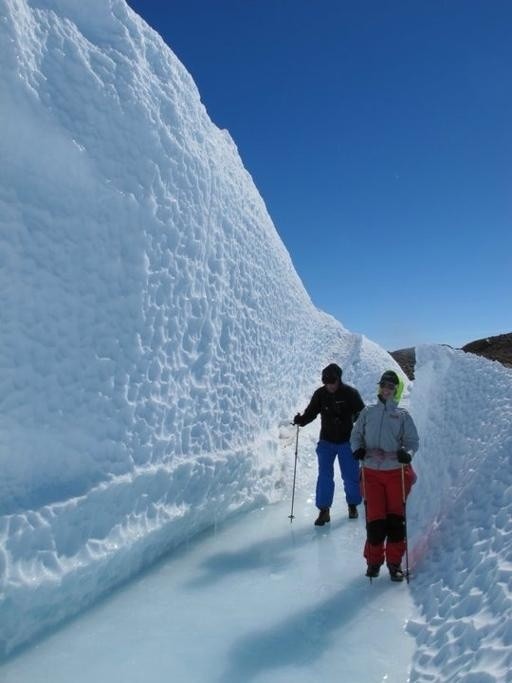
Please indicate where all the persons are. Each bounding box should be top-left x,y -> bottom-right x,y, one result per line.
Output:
294,362 -> 366,524
349,368 -> 418,583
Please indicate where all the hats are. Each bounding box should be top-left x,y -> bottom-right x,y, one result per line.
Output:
377,371 -> 399,386
321,363 -> 342,385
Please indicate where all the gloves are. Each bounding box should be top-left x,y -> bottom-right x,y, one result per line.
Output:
352,448 -> 366,460
396,450 -> 412,464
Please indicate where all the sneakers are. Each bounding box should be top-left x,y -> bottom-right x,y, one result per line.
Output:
314,508 -> 330,526
366,564 -> 380,578
388,563 -> 404,582
348,504 -> 358,518
